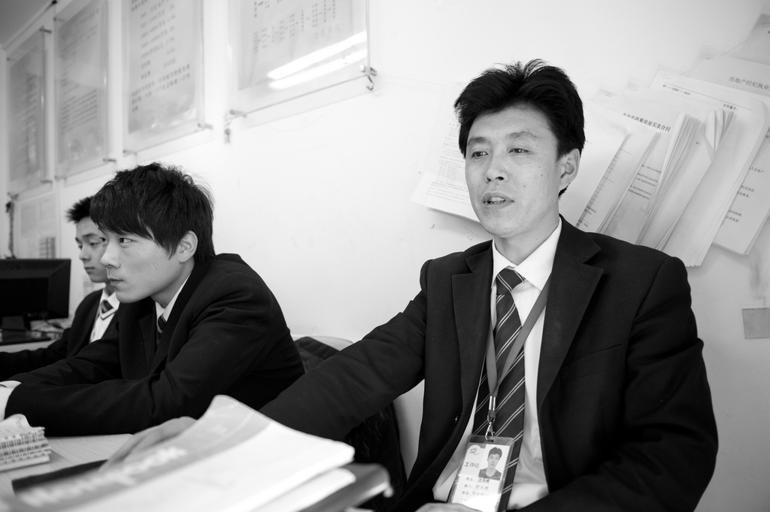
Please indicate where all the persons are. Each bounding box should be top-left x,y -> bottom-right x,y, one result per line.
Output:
98,55 -> 719,511
0,161 -> 305,437
0,195 -> 120,382
479,447 -> 503,480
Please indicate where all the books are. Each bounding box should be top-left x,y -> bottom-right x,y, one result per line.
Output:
0,394 -> 390,511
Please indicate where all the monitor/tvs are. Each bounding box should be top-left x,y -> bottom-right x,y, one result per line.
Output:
0,257 -> 72,345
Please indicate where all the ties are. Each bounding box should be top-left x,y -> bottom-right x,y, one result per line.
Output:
100,299 -> 112,313
156,313 -> 167,350
470,268 -> 526,512
